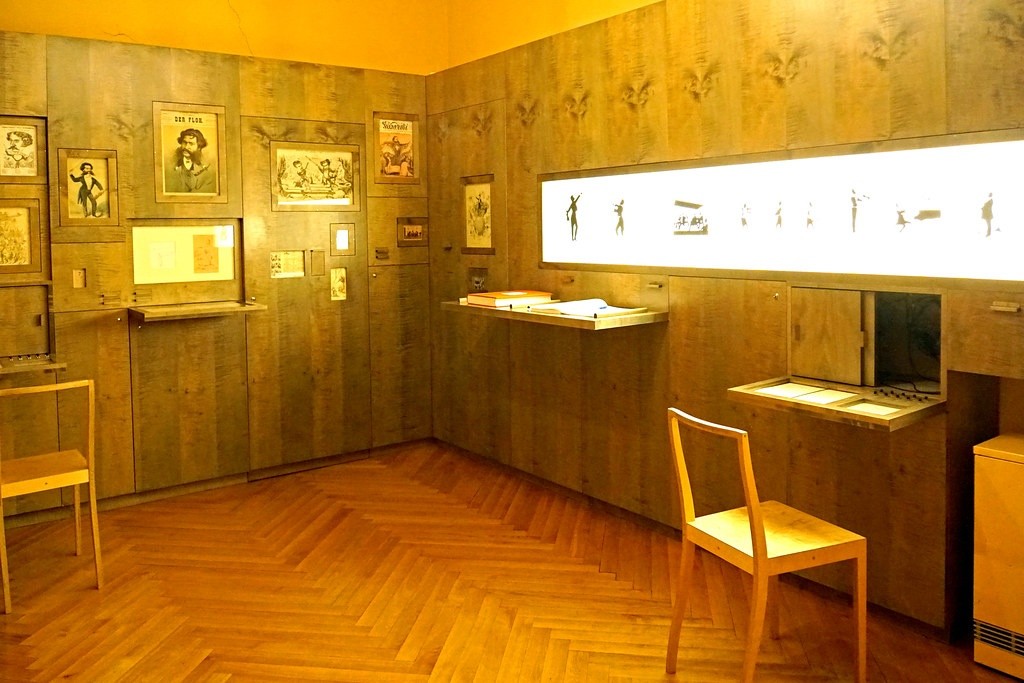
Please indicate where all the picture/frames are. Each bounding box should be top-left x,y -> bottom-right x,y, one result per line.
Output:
330,223 -> 356,256
460,175 -> 495,255
397,217 -> 429,247
374,110 -> 421,185
58,148 -> 119,227
269,140 -> 361,212
0,198 -> 41,274
153,101 -> 229,204
0,116 -> 48,185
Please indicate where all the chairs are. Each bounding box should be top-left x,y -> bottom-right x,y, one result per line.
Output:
0,379 -> 104,614
667,407 -> 868,683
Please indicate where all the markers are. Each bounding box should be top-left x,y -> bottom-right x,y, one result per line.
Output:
991,300 -> 1020,312
